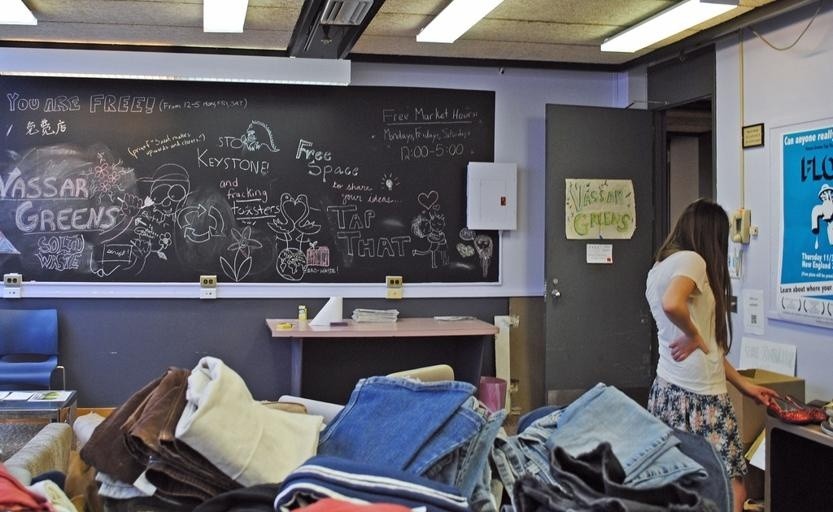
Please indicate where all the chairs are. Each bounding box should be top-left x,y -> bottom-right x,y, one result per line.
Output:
0,309 -> 66,390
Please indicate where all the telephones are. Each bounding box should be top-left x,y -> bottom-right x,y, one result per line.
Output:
729,209 -> 750,244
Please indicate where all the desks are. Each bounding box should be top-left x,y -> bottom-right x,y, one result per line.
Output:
765,416 -> 833,512
265,315 -> 501,398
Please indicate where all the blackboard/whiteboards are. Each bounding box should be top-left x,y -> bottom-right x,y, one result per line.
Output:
0,72 -> 502,287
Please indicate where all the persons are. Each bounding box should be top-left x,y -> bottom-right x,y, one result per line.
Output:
644,198 -> 785,512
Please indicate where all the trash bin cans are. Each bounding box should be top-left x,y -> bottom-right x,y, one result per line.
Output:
479,375 -> 506,412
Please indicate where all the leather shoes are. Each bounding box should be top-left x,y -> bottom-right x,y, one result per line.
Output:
767,393 -> 813,425
784,392 -> 829,424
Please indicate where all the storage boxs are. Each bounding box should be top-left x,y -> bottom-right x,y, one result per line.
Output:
727,368 -> 805,443
